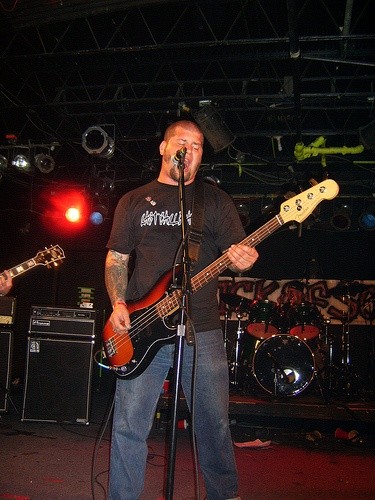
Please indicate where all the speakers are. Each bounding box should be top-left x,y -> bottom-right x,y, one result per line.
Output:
0,329 -> 15,414
21,337 -> 95,423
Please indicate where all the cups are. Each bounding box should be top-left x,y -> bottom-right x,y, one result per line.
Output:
160,380 -> 170,396
177,419 -> 187,428
335,428 -> 348,439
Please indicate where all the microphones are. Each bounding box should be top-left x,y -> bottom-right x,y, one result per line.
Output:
171,147 -> 186,164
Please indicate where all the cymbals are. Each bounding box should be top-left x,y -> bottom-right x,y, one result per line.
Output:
219,293 -> 252,306
329,314 -> 356,319
325,284 -> 373,295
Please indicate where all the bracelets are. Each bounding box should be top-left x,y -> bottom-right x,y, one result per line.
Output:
112,302 -> 128,309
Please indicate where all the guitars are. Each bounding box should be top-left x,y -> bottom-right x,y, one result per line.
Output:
0,244 -> 67,279
101,171 -> 340,381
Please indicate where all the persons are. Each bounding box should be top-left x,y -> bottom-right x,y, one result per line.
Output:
105,120 -> 258,500
264,280 -> 324,350
0,269 -> 13,296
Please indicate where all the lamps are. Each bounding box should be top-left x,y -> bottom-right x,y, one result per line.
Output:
196,104 -> 239,151
307,201 -> 375,233
82,122 -> 119,159
0,141 -> 55,173
261,197 -> 284,232
237,201 -> 252,226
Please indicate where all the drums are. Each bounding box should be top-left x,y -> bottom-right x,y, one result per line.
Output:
284,303 -> 321,341
250,334 -> 317,399
243,301 -> 281,340
